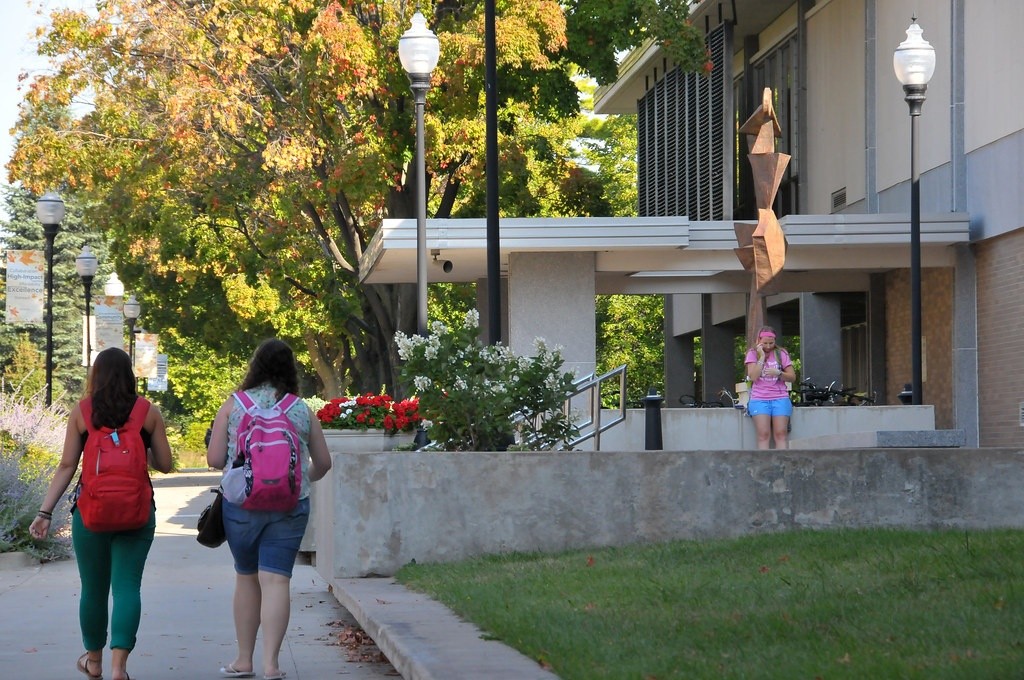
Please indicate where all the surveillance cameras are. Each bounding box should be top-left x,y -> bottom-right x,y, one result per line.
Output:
433,259 -> 454,273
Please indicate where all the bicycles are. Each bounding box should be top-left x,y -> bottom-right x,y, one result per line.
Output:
678,377 -> 875,408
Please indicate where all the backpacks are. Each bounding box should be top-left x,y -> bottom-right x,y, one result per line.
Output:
77,395 -> 151,531
232,391 -> 302,510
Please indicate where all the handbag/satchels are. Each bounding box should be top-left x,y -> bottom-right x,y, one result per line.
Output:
197,489 -> 226,549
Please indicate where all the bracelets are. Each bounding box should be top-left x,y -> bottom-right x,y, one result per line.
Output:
37,509 -> 53,521
777,370 -> 782,378
756,361 -> 764,366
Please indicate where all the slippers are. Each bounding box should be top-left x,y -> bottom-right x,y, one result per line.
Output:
220,664 -> 256,677
77,652 -> 103,680
264,671 -> 287,679
125,671 -> 136,680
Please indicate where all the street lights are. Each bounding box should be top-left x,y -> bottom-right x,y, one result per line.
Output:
892,13 -> 936,405
36,194 -> 65,409
398,5 -> 440,453
75,245 -> 97,366
104,273 -> 125,295
124,293 -> 141,358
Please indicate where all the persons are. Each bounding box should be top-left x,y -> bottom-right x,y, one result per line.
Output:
29,347 -> 174,680
743,325 -> 796,450
206,336 -> 333,679
